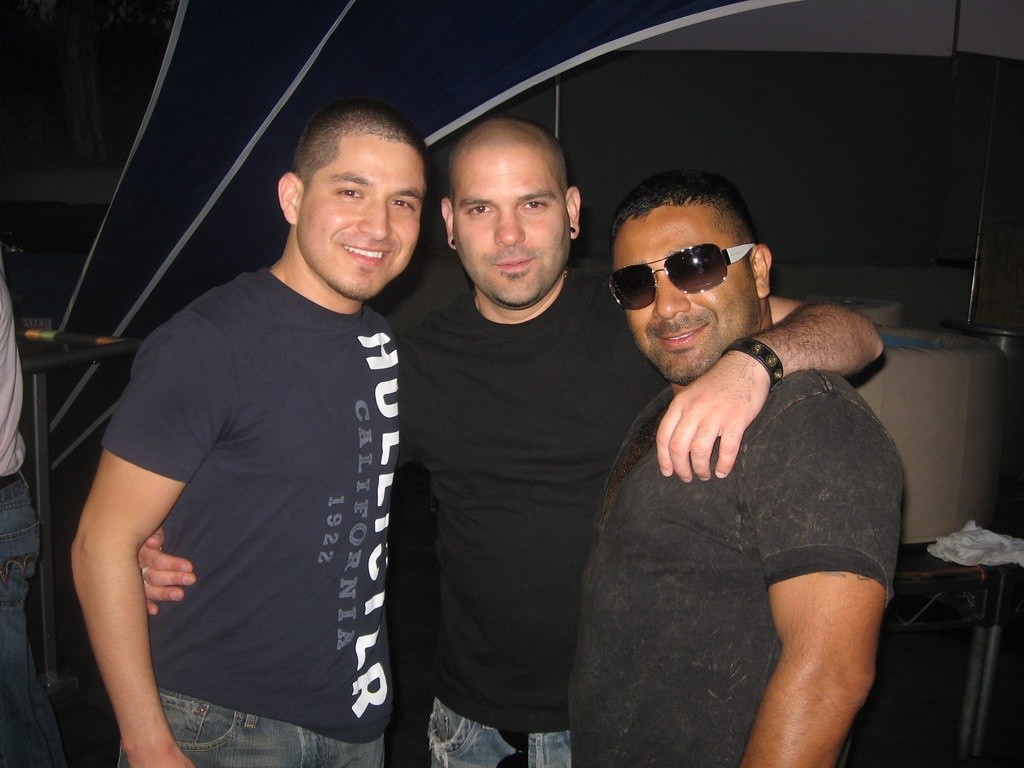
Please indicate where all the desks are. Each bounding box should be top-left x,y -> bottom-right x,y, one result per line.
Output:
880,548 -> 1012,760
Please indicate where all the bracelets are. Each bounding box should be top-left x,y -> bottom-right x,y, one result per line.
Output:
721,337 -> 783,391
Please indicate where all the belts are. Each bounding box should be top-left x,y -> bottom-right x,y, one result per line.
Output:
0,471 -> 23,490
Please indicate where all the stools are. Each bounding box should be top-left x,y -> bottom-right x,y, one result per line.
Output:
834,296 -> 1008,544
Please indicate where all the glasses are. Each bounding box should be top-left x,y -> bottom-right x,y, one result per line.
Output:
609,243 -> 756,311
495,728 -> 529,768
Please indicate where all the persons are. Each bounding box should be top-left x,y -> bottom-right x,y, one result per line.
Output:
72,98 -> 906,768
0,267 -> 63,768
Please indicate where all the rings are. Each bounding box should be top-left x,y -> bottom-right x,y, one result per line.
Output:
140,566 -> 149,585
